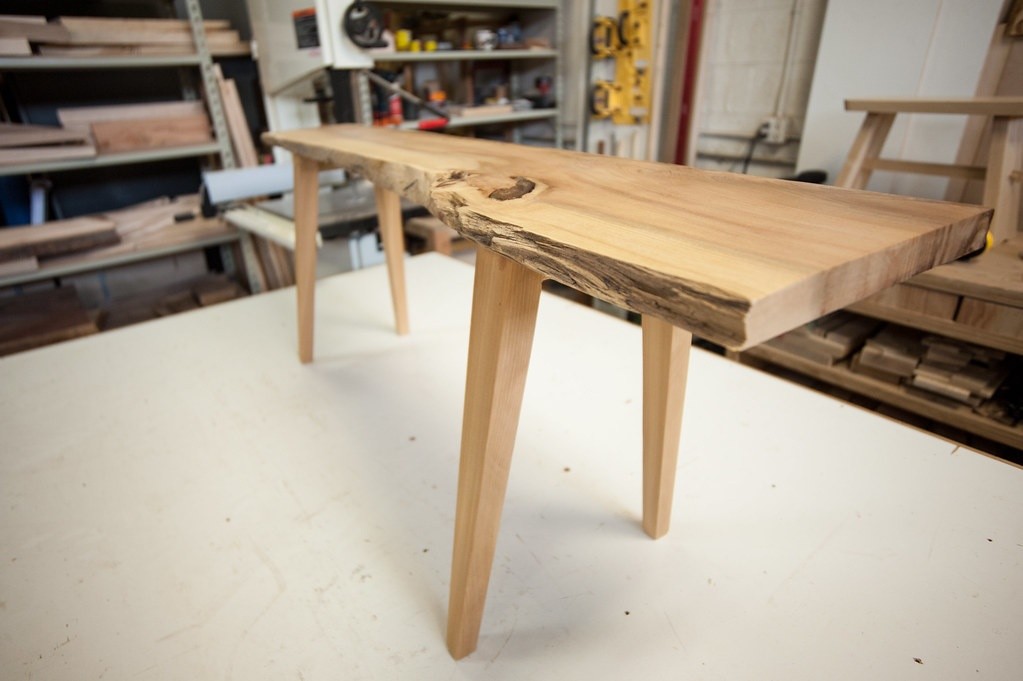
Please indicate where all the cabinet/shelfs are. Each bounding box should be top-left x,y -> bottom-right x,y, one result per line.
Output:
0,0 -> 271,359
247,0 -> 594,285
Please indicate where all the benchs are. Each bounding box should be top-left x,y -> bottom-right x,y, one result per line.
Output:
835,96 -> 1023,251
263,123 -> 992,660
